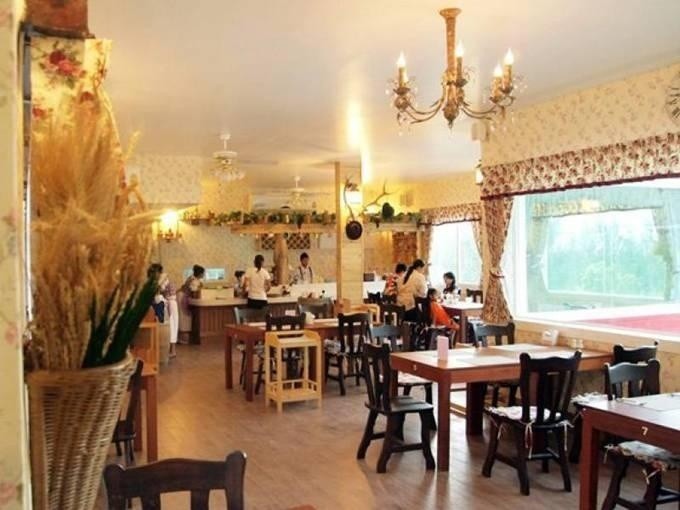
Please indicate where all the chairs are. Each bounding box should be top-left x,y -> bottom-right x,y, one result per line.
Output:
470,321 -> 520,410
109,302 -> 164,463
601,359 -> 680,510
567,340 -> 659,464
103,451 -> 247,509
356,336 -> 436,472
223,283 -> 488,412
481,350 -> 583,495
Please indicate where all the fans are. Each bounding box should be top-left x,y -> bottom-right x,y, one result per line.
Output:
262,174 -> 326,199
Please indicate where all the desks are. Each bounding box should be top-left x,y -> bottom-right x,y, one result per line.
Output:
187,282 -> 337,345
389,343 -> 614,472
581,392 -> 680,510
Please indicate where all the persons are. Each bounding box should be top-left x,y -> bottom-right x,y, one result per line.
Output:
148,264 -> 178,358
234,271 -> 246,298
243,255 -> 271,343
382,259 -> 466,349
176,265 -> 204,344
290,252 -> 315,287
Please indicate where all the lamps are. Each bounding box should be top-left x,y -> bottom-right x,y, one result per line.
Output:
205,131 -> 246,183
475,158 -> 485,187
386,5 -> 525,144
342,179 -> 364,241
